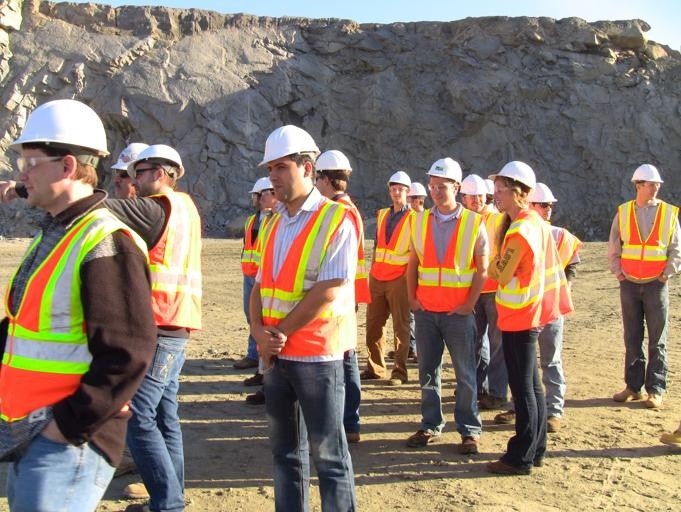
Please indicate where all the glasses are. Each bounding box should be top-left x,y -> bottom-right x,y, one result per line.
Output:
112,168 -> 131,179
14,153 -> 66,175
533,201 -> 556,209
135,166 -> 158,181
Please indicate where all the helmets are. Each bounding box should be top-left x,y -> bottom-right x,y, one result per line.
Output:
255,124 -> 322,170
387,170 -> 428,198
11,97 -> 112,160
458,173 -> 493,197
631,162 -> 666,185
112,142 -> 148,168
246,175 -> 275,194
528,181 -> 560,206
424,155 -> 464,185
488,159 -> 537,190
314,149 -> 354,175
132,141 -> 187,183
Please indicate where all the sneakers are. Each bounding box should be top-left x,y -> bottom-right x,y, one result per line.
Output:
346,432 -> 361,442
406,427 -> 442,449
613,385 -> 643,402
242,371 -> 267,388
230,355 -> 261,370
494,408 -> 517,423
481,453 -> 546,478
546,415 -> 562,433
112,456 -> 152,512
244,390 -> 267,408
645,391 -> 664,408
360,370 -> 409,385
386,342 -> 420,364
459,433 -> 480,456
452,381 -> 511,411
659,429 -> 681,447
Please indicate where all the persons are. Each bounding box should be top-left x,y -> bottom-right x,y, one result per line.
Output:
660,420 -> 681,449
235,125 -> 372,511
607,162 -> 681,407
0,99 -> 160,512
0,143 -> 204,511
361,157 -> 583,473
111,138 -> 150,199
